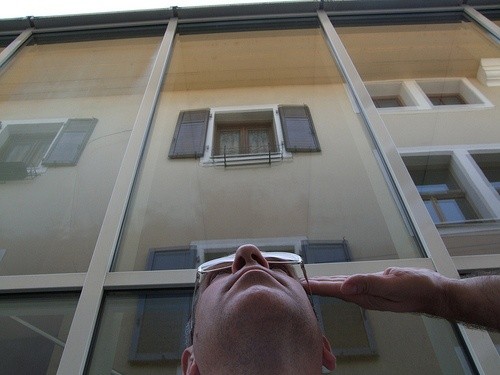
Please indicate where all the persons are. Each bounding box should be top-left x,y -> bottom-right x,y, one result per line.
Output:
182,244 -> 500,375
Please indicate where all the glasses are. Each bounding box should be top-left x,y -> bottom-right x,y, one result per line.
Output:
190,251 -> 318,336
